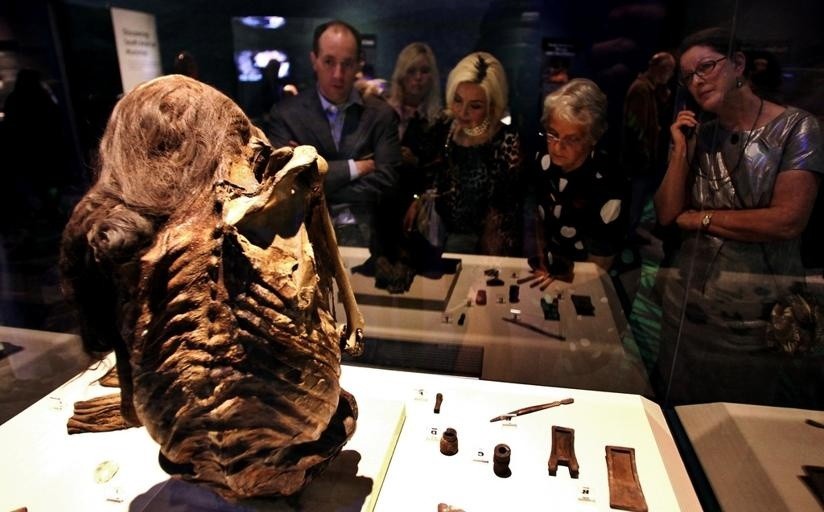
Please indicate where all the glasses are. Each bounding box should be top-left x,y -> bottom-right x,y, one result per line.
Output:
678,55 -> 732,86
539,132 -> 591,144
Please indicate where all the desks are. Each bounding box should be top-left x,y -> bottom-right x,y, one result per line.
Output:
335,244 -> 642,363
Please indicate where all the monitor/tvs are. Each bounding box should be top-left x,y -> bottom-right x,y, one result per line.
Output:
236,50 -> 289,84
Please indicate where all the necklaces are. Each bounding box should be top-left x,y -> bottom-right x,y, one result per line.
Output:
462,118 -> 488,136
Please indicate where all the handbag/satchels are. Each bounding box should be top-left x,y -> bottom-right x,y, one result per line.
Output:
769,290 -> 823,352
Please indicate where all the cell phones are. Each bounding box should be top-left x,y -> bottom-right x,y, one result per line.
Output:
681,95 -> 700,141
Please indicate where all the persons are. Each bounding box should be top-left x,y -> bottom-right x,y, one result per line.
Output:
383,42 -> 445,155
624,52 -> 675,185
623,28 -> 823,403
404,52 -> 526,258
522,77 -> 627,283
0,52 -> 60,129
268,21 -> 402,249
